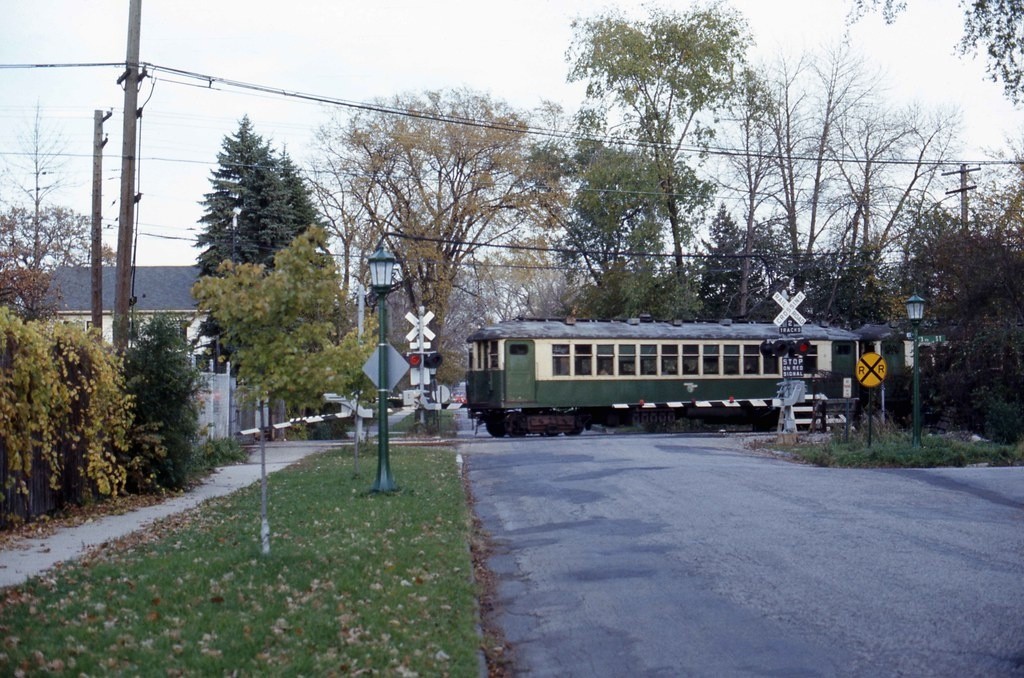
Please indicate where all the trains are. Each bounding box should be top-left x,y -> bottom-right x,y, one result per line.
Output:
465,314 -> 1024,437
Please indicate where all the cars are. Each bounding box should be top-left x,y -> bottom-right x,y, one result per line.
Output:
450,390 -> 467,404
388,392 -> 403,406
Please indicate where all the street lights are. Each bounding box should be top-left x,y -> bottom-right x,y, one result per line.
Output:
902,287 -> 926,448
370,244 -> 401,492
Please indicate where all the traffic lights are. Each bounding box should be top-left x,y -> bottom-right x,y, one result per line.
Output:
409,354 -> 421,368
772,340 -> 789,356
424,352 -> 443,369
794,339 -> 811,355
